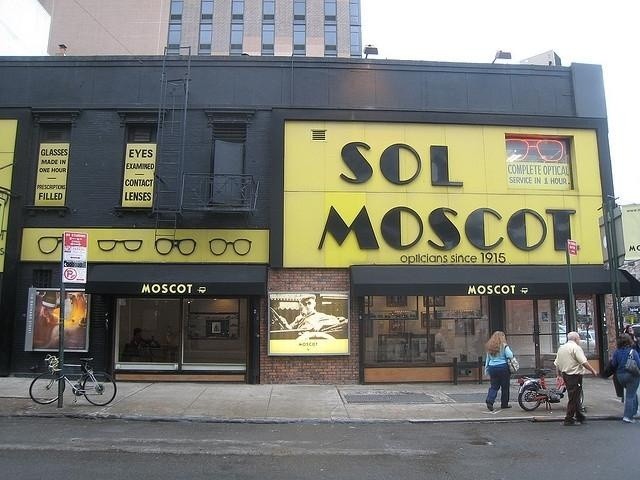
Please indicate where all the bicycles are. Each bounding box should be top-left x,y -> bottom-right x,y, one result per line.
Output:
28,354 -> 120,406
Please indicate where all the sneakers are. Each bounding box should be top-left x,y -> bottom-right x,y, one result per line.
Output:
501,405 -> 511,408
623,416 -> 634,423
486,401 -> 493,410
564,421 -> 580,426
633,411 -> 640,418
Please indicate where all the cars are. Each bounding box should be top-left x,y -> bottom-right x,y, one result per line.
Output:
559,324 -> 640,354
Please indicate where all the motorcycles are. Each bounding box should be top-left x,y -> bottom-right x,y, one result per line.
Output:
518,364 -> 584,413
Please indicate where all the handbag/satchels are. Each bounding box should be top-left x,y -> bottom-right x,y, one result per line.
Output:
507,357 -> 520,373
625,348 -> 639,374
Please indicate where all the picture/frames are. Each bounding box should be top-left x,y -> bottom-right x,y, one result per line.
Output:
266,290 -> 351,358
363,296 -> 475,363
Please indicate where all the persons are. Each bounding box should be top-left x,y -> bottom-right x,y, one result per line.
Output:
622,324 -> 640,352
607,333 -> 639,423
484,331 -> 516,410
552,332 -> 597,425
127,326 -> 152,362
278,293 -> 345,341
